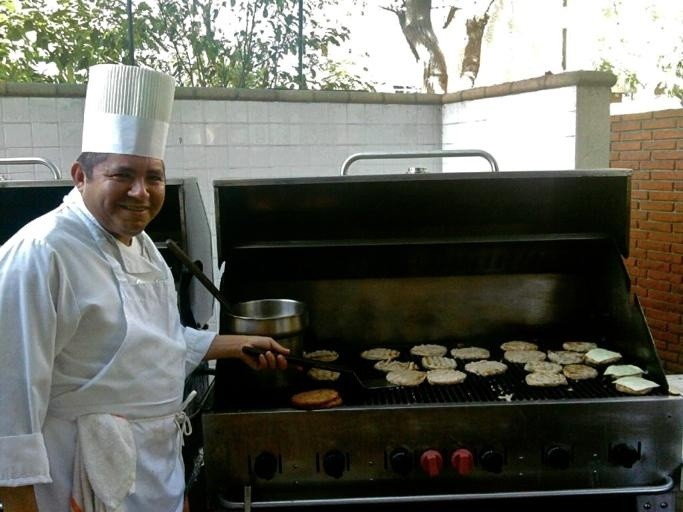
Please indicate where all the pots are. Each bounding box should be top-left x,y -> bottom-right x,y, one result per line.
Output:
223,297 -> 306,338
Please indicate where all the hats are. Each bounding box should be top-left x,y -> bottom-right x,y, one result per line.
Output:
80,63 -> 176,161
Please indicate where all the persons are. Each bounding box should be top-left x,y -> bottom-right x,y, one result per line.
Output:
0,154 -> 289,512
